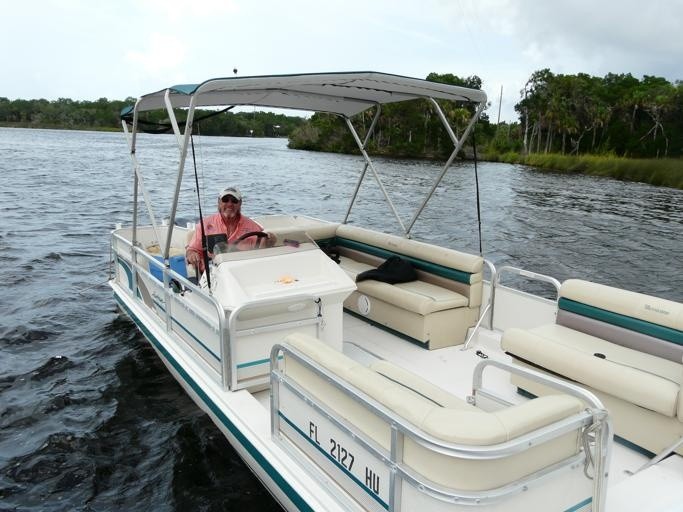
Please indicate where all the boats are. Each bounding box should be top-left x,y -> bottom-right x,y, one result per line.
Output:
96,65 -> 680,512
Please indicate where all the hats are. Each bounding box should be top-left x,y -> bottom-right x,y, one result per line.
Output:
219,185 -> 242,201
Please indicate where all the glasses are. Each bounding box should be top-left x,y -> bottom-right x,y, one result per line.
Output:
222,197 -> 239,205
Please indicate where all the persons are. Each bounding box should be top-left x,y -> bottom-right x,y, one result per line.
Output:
184,186 -> 276,276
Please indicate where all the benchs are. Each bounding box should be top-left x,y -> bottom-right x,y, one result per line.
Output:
330,226 -> 484,351
501,275 -> 683,463
288,333 -> 586,495
